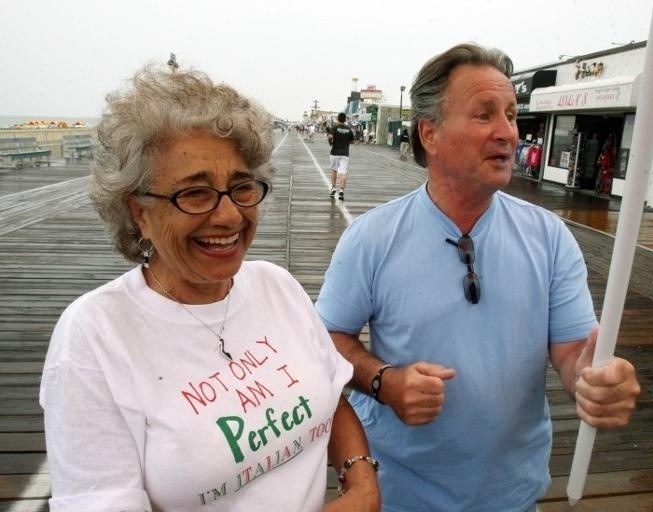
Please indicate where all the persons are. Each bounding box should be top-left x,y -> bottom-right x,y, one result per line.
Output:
35,63 -> 381,511
397,129 -> 409,161
314,41 -> 640,512
326,113 -> 354,197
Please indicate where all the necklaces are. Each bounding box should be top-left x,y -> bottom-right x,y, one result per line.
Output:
147,268 -> 231,360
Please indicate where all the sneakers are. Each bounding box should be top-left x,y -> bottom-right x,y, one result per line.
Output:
330,188 -> 336,195
339,190 -> 344,200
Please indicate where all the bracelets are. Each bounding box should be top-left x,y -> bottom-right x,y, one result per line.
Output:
334,454 -> 379,496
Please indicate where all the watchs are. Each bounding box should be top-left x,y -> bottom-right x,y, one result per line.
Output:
371,364 -> 393,404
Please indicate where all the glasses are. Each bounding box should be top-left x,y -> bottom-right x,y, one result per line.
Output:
131,178 -> 270,215
444,233 -> 482,305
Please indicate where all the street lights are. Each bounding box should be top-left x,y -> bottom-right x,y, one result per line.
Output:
399,86 -> 406,118
